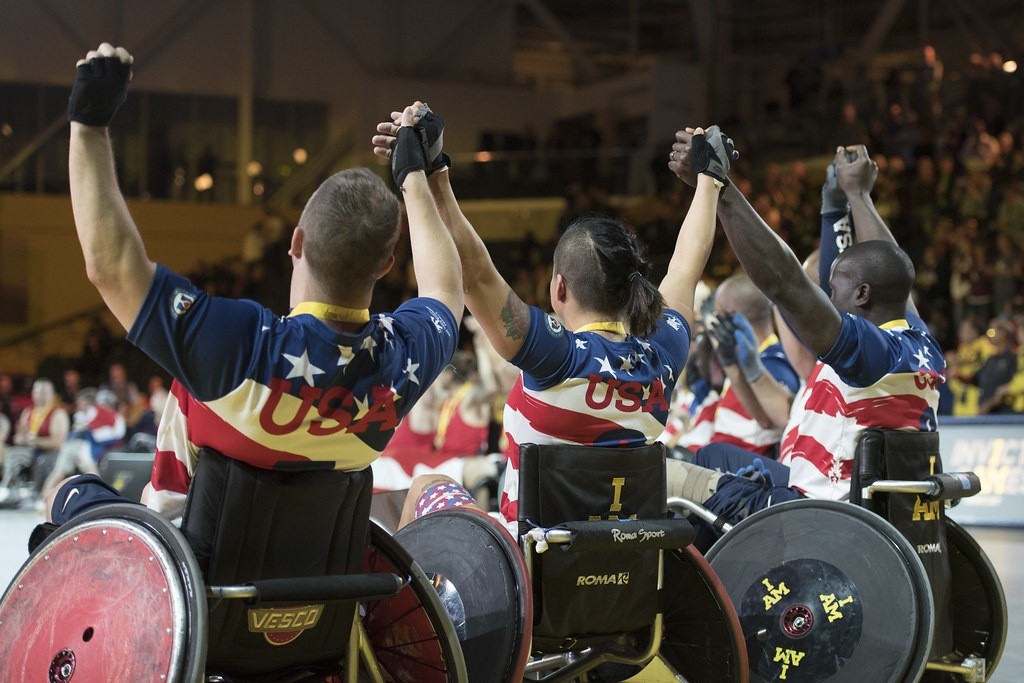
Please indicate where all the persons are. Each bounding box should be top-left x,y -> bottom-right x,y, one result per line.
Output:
45,42 -> 465,531
396,101 -> 731,544
664,124 -> 948,559
2,43 -> 1024,510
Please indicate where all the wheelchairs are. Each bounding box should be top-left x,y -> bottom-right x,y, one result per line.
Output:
0,428 -> 1009,683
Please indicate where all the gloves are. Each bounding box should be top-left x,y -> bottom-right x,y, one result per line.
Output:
733,312 -> 765,382
710,311 -> 737,366
820,152 -> 858,214
736,458 -> 775,488
